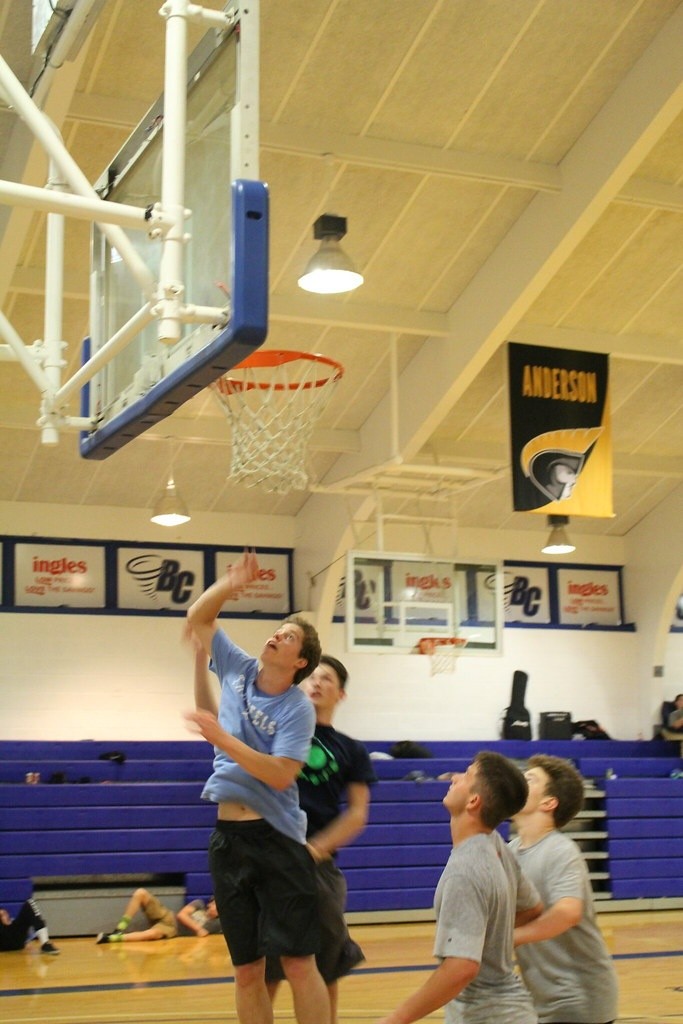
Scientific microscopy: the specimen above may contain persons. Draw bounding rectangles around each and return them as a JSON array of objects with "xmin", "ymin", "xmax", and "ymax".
[
  {"xmin": 191, "ymin": 628, "xmax": 371, "ymax": 1024},
  {"xmin": 187, "ymin": 548, "xmax": 334, "ymax": 1024},
  {"xmin": 382, "ymin": 753, "xmax": 543, "ymax": 1024},
  {"xmin": 95, "ymin": 888, "xmax": 222, "ymax": 943},
  {"xmin": 0, "ymin": 899, "xmax": 60, "ymax": 955},
  {"xmin": 504, "ymin": 755, "xmax": 621, "ymax": 1024}
]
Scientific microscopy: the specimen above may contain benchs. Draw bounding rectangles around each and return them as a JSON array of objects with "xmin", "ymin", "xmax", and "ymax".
[{"xmin": 1, "ymin": 739, "xmax": 683, "ymax": 939}]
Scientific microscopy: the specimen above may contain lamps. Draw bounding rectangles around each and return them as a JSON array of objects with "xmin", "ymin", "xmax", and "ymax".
[
  {"xmin": 296, "ymin": 213, "xmax": 366, "ymax": 296},
  {"xmin": 541, "ymin": 514, "xmax": 577, "ymax": 555},
  {"xmin": 149, "ymin": 435, "xmax": 191, "ymax": 527}
]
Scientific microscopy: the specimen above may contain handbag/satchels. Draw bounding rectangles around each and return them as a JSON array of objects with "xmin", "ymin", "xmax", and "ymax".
[{"xmin": 538, "ymin": 711, "xmax": 573, "ymax": 739}]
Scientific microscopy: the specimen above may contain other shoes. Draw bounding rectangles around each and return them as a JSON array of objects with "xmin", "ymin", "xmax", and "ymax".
[
  {"xmin": 96, "ymin": 932, "xmax": 109, "ymax": 945},
  {"xmin": 110, "ymin": 929, "xmax": 123, "ymax": 935},
  {"xmin": 41, "ymin": 941, "xmax": 60, "ymax": 955}
]
